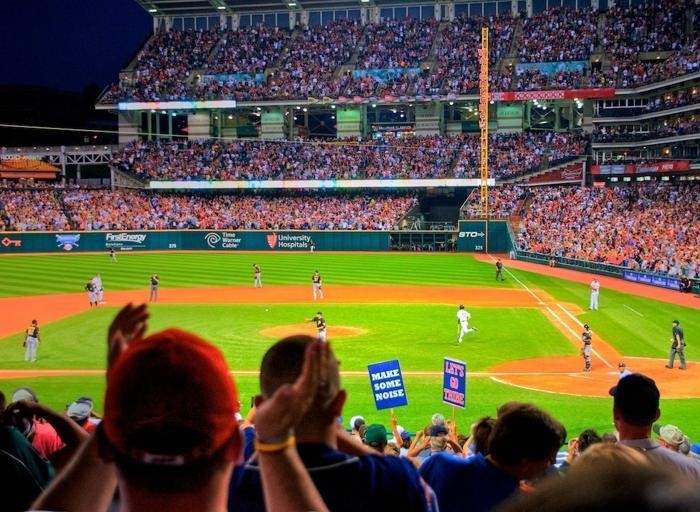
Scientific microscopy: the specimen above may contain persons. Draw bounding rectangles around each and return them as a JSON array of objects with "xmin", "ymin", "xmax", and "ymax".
[
  {"xmin": 312, "ymin": 270, "xmax": 324, "ymax": 300},
  {"xmin": 110, "ymin": 246, "xmax": 118, "ymax": 264},
  {"xmin": 1, "ymin": 177, "xmax": 420, "ymax": 231},
  {"xmin": 309, "ymin": 239, "xmax": 315, "ymax": 252},
  {"xmin": 1, "ymin": 303, "xmax": 700, "ymax": 510},
  {"xmin": 148, "ymin": 271, "xmax": 159, "ymax": 302},
  {"xmin": 448, "ymin": 176, "xmax": 700, "ymax": 311},
  {"xmin": 85, "ymin": 273, "xmax": 105, "ymax": 308},
  {"xmin": 101, "ymin": 1, "xmax": 699, "ymax": 178},
  {"xmin": 252, "ymin": 264, "xmax": 263, "ymax": 289}
]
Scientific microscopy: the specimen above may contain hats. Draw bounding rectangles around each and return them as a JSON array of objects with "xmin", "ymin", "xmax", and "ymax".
[
  {"xmin": 102, "ymin": 330, "xmax": 240, "ymax": 466},
  {"xmin": 608, "ymin": 374, "xmax": 660, "ymax": 410},
  {"xmin": 366, "ymin": 424, "xmax": 387, "ymax": 447},
  {"xmin": 67, "ymin": 398, "xmax": 93, "ymax": 421},
  {"xmin": 426, "ymin": 425, "xmax": 448, "ymax": 436},
  {"xmin": 653, "ymin": 424, "xmax": 685, "ymax": 446}
]
